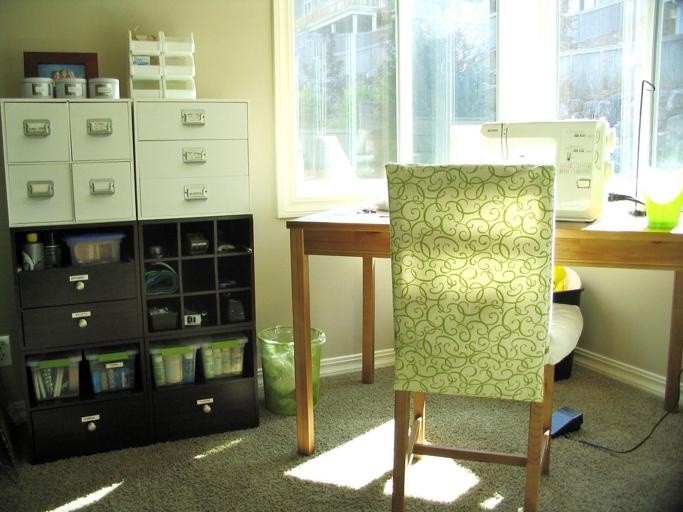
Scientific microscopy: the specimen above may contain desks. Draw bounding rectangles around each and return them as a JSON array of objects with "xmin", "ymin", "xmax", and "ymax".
[{"xmin": 286, "ymin": 211, "xmax": 683, "ymax": 457}]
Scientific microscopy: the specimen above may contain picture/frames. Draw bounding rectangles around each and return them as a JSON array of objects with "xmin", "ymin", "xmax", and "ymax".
[{"xmin": 23, "ymin": 53, "xmax": 99, "ymax": 80}]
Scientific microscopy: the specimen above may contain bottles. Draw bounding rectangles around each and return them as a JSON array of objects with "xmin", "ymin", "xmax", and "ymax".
[
  {"xmin": 151, "ymin": 346, "xmax": 243, "ymax": 386},
  {"xmin": 19, "ymin": 231, "xmax": 46, "ymax": 271}
]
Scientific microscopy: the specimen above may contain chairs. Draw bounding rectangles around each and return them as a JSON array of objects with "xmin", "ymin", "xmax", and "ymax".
[{"xmin": 385, "ymin": 163, "xmax": 555, "ymax": 512}]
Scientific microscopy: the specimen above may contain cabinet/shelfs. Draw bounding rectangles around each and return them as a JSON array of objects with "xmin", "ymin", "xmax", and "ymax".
[{"xmin": 128, "ymin": 30, "xmax": 196, "ymax": 99}]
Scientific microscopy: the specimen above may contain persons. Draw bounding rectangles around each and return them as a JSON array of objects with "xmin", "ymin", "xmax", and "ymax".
[{"xmin": 50, "ymin": 69, "xmax": 77, "ymax": 99}]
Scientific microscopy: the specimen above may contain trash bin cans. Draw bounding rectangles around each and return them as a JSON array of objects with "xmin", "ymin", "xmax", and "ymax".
[{"xmin": 257, "ymin": 326, "xmax": 326, "ymax": 415}]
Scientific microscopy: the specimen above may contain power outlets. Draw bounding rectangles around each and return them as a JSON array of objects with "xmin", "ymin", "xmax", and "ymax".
[{"xmin": 0, "ymin": 336, "xmax": 10, "ymax": 367}]
